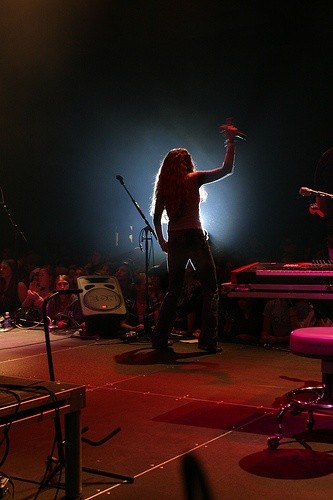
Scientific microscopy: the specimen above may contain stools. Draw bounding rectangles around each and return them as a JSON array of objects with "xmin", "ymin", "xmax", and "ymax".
[{"xmin": 268, "ymin": 326, "xmax": 333, "ymax": 453}]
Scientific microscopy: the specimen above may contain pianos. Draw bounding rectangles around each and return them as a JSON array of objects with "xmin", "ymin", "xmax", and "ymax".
[{"xmin": 220, "ymin": 260, "xmax": 333, "ymax": 325}]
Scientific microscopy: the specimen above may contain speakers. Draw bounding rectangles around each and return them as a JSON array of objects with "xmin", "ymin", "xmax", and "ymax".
[{"xmin": 76, "ymin": 275, "xmax": 128, "ymax": 314}]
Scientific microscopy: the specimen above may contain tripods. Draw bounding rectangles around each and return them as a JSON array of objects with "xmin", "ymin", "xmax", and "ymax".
[
  {"xmin": 121, "ymin": 183, "xmax": 185, "ymax": 340},
  {"xmin": 38, "ymin": 292, "xmax": 135, "ymax": 491}
]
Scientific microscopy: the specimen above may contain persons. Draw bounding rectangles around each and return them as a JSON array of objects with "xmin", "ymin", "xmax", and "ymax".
[
  {"xmin": 151, "ymin": 124, "xmax": 238, "ymax": 355},
  {"xmin": 0, "ymin": 249, "xmax": 333, "ymax": 346}
]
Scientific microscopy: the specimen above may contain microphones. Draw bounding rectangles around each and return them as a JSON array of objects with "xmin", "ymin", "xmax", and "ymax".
[
  {"xmin": 299, "ymin": 187, "xmax": 333, "ymax": 199},
  {"xmin": 235, "ymin": 132, "xmax": 247, "ymax": 141},
  {"xmin": 52, "ymin": 289, "xmax": 84, "ymax": 294},
  {"xmin": 116, "ymin": 175, "xmax": 123, "ymax": 182}
]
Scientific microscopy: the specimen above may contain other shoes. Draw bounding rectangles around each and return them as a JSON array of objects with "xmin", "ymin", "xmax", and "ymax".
[
  {"xmin": 198, "ymin": 345, "xmax": 224, "ymax": 352},
  {"xmin": 152, "ymin": 345, "xmax": 173, "ymax": 353}
]
